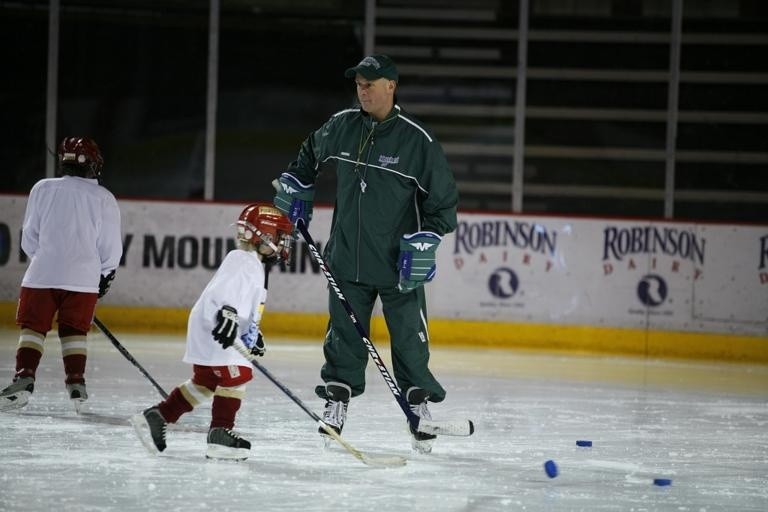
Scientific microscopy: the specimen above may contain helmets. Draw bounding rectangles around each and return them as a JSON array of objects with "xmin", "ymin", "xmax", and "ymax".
[
  {"xmin": 236, "ymin": 202, "xmax": 300, "ymax": 267},
  {"xmin": 56, "ymin": 134, "xmax": 106, "ymax": 179}
]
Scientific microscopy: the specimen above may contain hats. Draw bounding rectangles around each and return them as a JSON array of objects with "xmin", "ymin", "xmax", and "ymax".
[{"xmin": 344, "ymin": 54, "xmax": 402, "ymax": 81}]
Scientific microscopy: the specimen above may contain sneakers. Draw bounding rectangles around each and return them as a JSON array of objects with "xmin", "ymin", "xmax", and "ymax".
[
  {"xmin": 406, "ymin": 386, "xmax": 438, "ymax": 443},
  {"xmin": 0, "ymin": 376, "xmax": 35, "ymax": 396},
  {"xmin": 65, "ymin": 382, "xmax": 88, "ymax": 401},
  {"xmin": 141, "ymin": 404, "xmax": 168, "ymax": 452},
  {"xmin": 207, "ymin": 426, "xmax": 250, "ymax": 448},
  {"xmin": 318, "ymin": 381, "xmax": 352, "ymax": 440}
]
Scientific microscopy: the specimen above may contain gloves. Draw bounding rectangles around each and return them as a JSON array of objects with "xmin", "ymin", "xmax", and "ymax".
[
  {"xmin": 210, "ymin": 304, "xmax": 241, "ymax": 351},
  {"xmin": 271, "ymin": 173, "xmax": 316, "ymax": 242},
  {"xmin": 396, "ymin": 231, "xmax": 442, "ymax": 294},
  {"xmin": 241, "ymin": 329, "xmax": 268, "ymax": 357},
  {"xmin": 97, "ymin": 270, "xmax": 116, "ymax": 300}
]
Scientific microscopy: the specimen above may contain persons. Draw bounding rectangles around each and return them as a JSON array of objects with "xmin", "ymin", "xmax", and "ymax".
[
  {"xmin": 143, "ymin": 201, "xmax": 295, "ymax": 454},
  {"xmin": 272, "ymin": 54, "xmax": 459, "ymax": 441},
  {"xmin": 0, "ymin": 135, "xmax": 123, "ymax": 400}
]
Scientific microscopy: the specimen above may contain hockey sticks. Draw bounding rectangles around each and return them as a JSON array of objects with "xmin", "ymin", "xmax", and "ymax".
[
  {"xmin": 236, "ymin": 339, "xmax": 405, "ymax": 468},
  {"xmin": 295, "ymin": 217, "xmax": 474, "ymax": 438}
]
